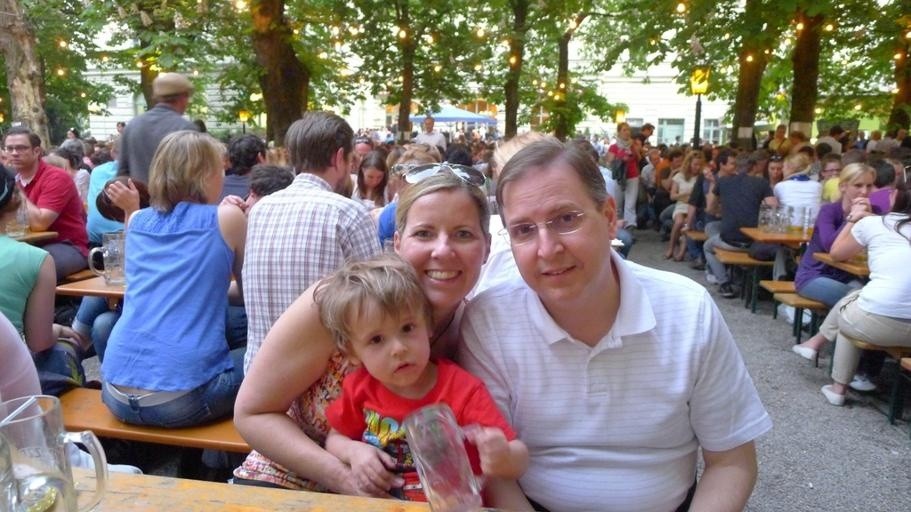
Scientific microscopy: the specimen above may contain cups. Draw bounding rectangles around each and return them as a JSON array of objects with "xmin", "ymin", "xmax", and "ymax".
[
  {"xmin": 0, "ymin": 395, "xmax": 110, "ymax": 512},
  {"xmin": 87, "ymin": 232, "xmax": 126, "ymax": 287},
  {"xmin": 405, "ymin": 400, "xmax": 492, "ymax": 512},
  {"xmin": 759, "ymin": 203, "xmax": 813, "ymax": 235},
  {"xmin": 383, "ymin": 237, "xmax": 395, "ymax": 255},
  {"xmin": 5, "ymin": 200, "xmax": 27, "ymax": 237},
  {"xmin": 855, "ymin": 248, "xmax": 868, "ymax": 264}
]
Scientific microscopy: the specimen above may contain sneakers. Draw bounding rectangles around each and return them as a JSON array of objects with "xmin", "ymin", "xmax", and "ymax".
[
  {"xmin": 822, "ymin": 384, "xmax": 845, "ymax": 407},
  {"xmin": 689, "ymin": 259, "xmax": 706, "ymax": 270},
  {"xmin": 792, "ymin": 344, "xmax": 817, "ymax": 360},
  {"xmin": 719, "ymin": 286, "xmax": 734, "ymax": 297},
  {"xmin": 849, "ymin": 374, "xmax": 877, "ymax": 392}
]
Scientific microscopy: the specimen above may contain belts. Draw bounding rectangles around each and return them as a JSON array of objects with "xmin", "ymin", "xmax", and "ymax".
[{"xmin": 105, "ymin": 381, "xmax": 188, "ymax": 407}]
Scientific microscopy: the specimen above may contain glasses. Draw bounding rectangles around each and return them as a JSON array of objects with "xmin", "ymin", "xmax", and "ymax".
[
  {"xmin": 769, "ymin": 156, "xmax": 784, "ymax": 160},
  {"xmin": 498, "ymin": 206, "xmax": 599, "ymax": 246},
  {"xmin": 403, "ymin": 162, "xmax": 486, "ymax": 188}
]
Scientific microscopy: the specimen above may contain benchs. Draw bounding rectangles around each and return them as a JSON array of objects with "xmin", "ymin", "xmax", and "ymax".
[
  {"xmin": 1, "ymin": 157, "xmax": 621, "ymax": 512},
  {"xmin": 684, "ymin": 188, "xmax": 911, "ymax": 424}
]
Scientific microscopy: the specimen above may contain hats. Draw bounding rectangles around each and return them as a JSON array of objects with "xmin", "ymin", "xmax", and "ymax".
[{"xmin": 151, "ymin": 72, "xmax": 193, "ymax": 97}]
